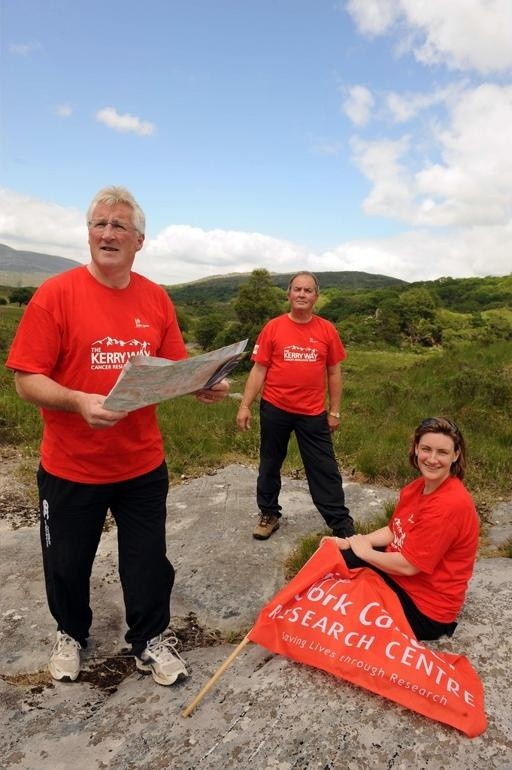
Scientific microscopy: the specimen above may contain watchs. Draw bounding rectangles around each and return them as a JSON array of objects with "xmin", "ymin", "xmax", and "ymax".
[{"xmin": 328, "ymin": 411, "xmax": 340, "ymax": 419}]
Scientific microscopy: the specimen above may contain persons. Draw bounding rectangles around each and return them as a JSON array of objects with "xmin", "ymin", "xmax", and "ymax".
[
  {"xmin": 316, "ymin": 414, "xmax": 482, "ymax": 643},
  {"xmin": 4, "ymin": 182, "xmax": 229, "ymax": 684},
  {"xmin": 234, "ymin": 270, "xmax": 359, "ymax": 543}
]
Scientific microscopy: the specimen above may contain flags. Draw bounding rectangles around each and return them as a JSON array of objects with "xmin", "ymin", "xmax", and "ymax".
[{"xmin": 248, "ymin": 538, "xmax": 488, "ymax": 738}]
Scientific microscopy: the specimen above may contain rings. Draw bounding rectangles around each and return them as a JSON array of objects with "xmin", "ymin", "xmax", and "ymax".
[{"xmin": 214, "ymin": 396, "xmax": 217, "ymax": 401}]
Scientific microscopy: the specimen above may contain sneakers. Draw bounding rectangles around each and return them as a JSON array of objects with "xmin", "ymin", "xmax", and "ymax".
[
  {"xmin": 252, "ymin": 512, "xmax": 281, "ymax": 540},
  {"xmin": 47, "ymin": 631, "xmax": 82, "ymax": 682},
  {"xmin": 135, "ymin": 635, "xmax": 188, "ymax": 686}
]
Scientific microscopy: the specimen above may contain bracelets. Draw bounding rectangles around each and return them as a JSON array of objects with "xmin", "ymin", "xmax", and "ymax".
[{"xmin": 238, "ymin": 406, "xmax": 249, "ymax": 409}]
